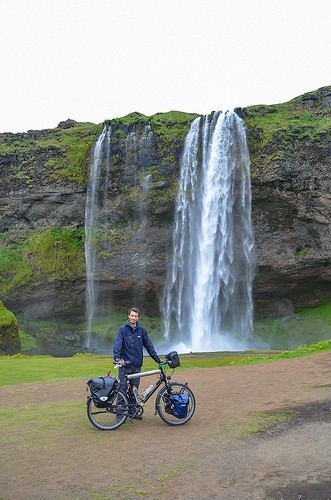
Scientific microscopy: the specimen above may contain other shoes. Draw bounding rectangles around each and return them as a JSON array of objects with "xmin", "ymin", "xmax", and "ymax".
[
  {"xmin": 130, "ymin": 414, "xmax": 142, "ymax": 420},
  {"xmin": 117, "ymin": 417, "xmax": 125, "ymax": 423}
]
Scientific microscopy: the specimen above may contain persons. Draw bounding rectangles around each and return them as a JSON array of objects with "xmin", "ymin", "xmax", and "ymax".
[{"xmin": 113, "ymin": 307, "xmax": 163, "ymax": 422}]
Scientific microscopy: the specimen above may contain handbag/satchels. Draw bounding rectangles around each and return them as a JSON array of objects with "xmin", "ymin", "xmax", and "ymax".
[
  {"xmin": 88, "ymin": 376, "xmax": 117, "ymax": 408},
  {"xmin": 163, "ymin": 392, "xmax": 189, "ymax": 419},
  {"xmin": 165, "ymin": 351, "xmax": 180, "ymax": 368}
]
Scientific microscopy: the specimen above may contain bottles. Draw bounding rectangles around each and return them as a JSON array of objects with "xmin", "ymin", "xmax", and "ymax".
[
  {"xmin": 132, "ymin": 385, "xmax": 142, "ymax": 403},
  {"xmin": 141, "ymin": 384, "xmax": 155, "ymax": 397}
]
